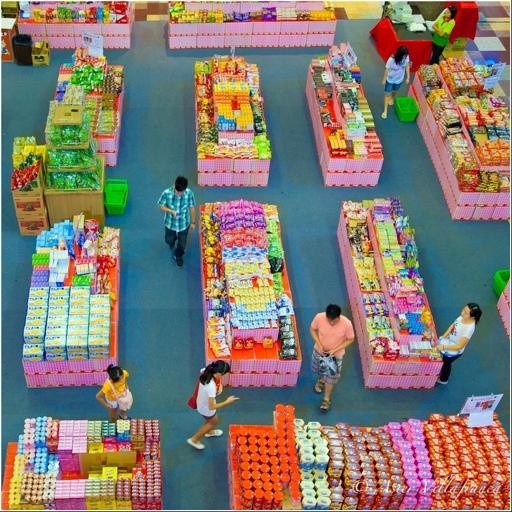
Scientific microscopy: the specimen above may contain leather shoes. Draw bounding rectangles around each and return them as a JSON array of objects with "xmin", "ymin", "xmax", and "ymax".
[
  {"xmin": 176, "ymin": 256, "xmax": 182, "ymax": 265},
  {"xmin": 119, "ymin": 416, "xmax": 131, "ymax": 420}
]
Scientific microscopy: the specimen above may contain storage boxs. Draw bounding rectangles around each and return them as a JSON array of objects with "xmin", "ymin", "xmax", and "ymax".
[{"xmin": 9, "ymin": 135, "xmax": 49, "ymax": 237}]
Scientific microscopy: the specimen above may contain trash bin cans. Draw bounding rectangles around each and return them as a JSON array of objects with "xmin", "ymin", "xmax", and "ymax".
[{"xmin": 13, "ymin": 34, "xmax": 33, "ymax": 65}]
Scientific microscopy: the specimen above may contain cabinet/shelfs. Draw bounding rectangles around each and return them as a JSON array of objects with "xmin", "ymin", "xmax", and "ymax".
[{"xmin": 44, "ymin": 104, "xmax": 107, "ymax": 226}]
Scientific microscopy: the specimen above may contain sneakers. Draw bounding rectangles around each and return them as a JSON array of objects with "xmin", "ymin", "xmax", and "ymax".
[
  {"xmin": 204, "ymin": 429, "xmax": 223, "ymax": 437},
  {"xmin": 186, "ymin": 438, "xmax": 205, "ymax": 450}
]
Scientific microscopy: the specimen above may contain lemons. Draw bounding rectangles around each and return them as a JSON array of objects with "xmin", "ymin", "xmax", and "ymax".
[
  {"xmin": 175, "ymin": 9, "xmax": 224, "ymax": 24},
  {"xmin": 309, "ymin": 8, "xmax": 335, "ymax": 20}
]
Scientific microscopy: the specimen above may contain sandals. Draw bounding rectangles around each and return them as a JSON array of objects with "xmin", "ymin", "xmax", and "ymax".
[
  {"xmin": 320, "ymin": 399, "xmax": 331, "ymax": 413},
  {"xmin": 314, "ymin": 380, "xmax": 325, "ymax": 393}
]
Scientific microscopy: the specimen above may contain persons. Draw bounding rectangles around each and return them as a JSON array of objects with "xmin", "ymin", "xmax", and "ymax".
[
  {"xmin": 430, "ymin": 4, "xmax": 458, "ymax": 65},
  {"xmin": 186, "ymin": 359, "xmax": 240, "ymax": 450},
  {"xmin": 381, "ymin": 45, "xmax": 410, "ymax": 120},
  {"xmin": 309, "ymin": 303, "xmax": 355, "ymax": 414},
  {"xmin": 95, "ymin": 364, "xmax": 134, "ymax": 422},
  {"xmin": 437, "ymin": 302, "xmax": 482, "ymax": 385},
  {"xmin": 156, "ymin": 175, "xmax": 197, "ymax": 268}
]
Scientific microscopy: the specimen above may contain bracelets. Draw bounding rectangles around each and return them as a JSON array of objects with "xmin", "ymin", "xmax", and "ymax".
[
  {"xmin": 191, "ymin": 221, "xmax": 195, "ymax": 224},
  {"xmin": 443, "ymin": 343, "xmax": 447, "ymax": 350}
]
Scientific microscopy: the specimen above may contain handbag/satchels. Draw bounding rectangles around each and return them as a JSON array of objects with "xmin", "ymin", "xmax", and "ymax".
[
  {"xmin": 188, "ymin": 382, "xmax": 199, "ymax": 409},
  {"xmin": 118, "ymin": 389, "xmax": 133, "ymax": 410}
]
